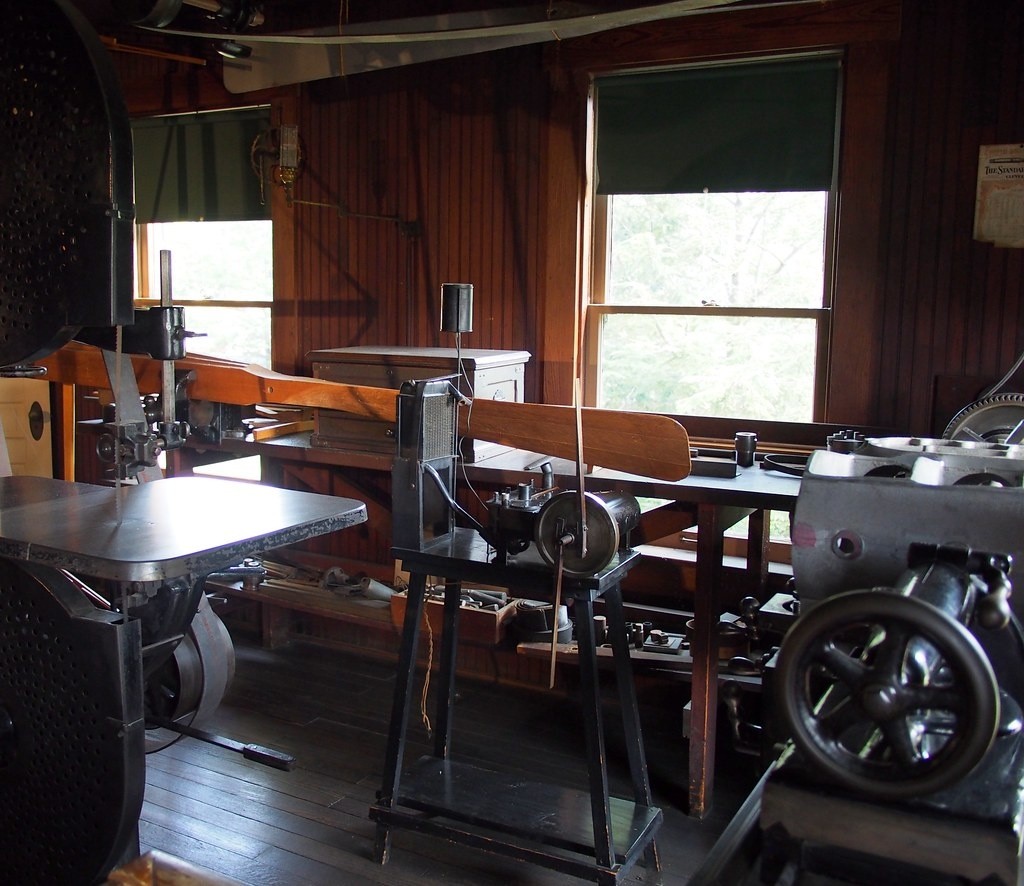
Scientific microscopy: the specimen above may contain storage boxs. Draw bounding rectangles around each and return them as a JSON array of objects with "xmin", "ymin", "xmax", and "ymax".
[{"xmin": 390, "ymin": 586, "xmax": 518, "ymax": 644}]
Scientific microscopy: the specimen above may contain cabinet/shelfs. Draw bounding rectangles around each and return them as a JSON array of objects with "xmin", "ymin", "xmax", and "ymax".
[{"xmin": 307, "ymin": 345, "xmax": 532, "ymax": 465}]
